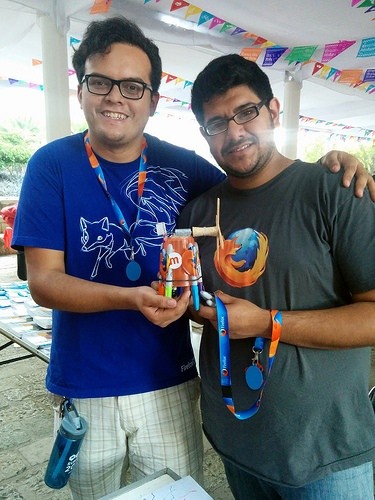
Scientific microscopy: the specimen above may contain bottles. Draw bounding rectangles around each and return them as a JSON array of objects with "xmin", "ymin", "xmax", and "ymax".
[{"xmin": 44, "ymin": 402, "xmax": 88, "ymax": 490}]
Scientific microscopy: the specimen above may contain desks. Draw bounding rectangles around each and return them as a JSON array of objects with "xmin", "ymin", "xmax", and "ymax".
[{"xmin": 0, "ymin": 282, "xmax": 202, "ymax": 378}]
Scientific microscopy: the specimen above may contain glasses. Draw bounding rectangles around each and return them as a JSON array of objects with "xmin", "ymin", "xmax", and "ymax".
[
  {"xmin": 201, "ymin": 97, "xmax": 269, "ymax": 137},
  {"xmin": 81, "ymin": 74, "xmax": 153, "ymax": 100}
]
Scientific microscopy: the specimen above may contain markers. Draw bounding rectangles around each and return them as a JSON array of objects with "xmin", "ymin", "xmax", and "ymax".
[
  {"xmin": 158, "ymin": 242, "xmax": 173, "ymax": 299},
  {"xmin": 187, "ymin": 243, "xmax": 204, "ymax": 312},
  {"xmin": 199, "ymin": 289, "xmax": 216, "ymax": 307}
]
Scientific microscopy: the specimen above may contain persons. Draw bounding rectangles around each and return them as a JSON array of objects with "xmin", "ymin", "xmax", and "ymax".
[
  {"xmin": 10, "ymin": 17, "xmax": 374, "ymax": 500},
  {"xmin": 148, "ymin": 55, "xmax": 375, "ymax": 500}
]
